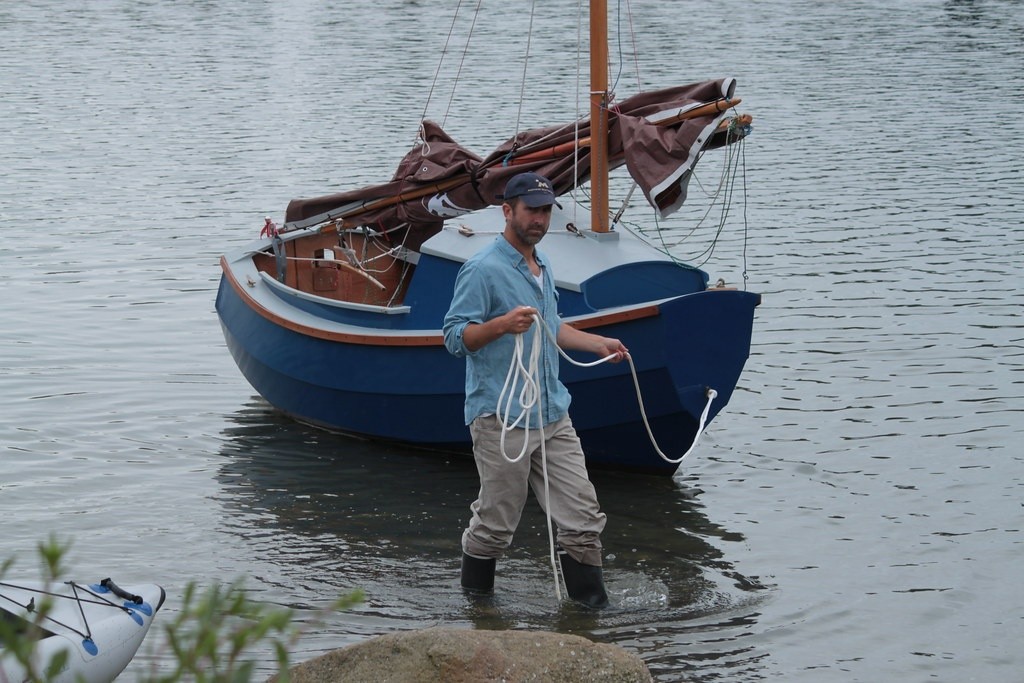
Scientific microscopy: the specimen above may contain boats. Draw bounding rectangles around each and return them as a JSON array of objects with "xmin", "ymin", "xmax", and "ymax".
[{"xmin": 214, "ymin": 0, "xmax": 765, "ymax": 483}]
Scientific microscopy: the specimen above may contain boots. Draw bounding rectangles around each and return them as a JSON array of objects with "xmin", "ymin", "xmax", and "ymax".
[
  {"xmin": 556, "ymin": 545, "xmax": 610, "ymax": 610},
  {"xmin": 459, "ymin": 552, "xmax": 497, "ymax": 590}
]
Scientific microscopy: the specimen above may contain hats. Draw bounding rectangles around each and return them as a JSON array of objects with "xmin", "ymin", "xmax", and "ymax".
[{"xmin": 504, "ymin": 171, "xmax": 563, "ymax": 211}]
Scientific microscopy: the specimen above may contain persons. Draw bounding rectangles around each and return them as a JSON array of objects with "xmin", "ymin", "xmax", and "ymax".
[{"xmin": 439, "ymin": 168, "xmax": 638, "ymax": 623}]
